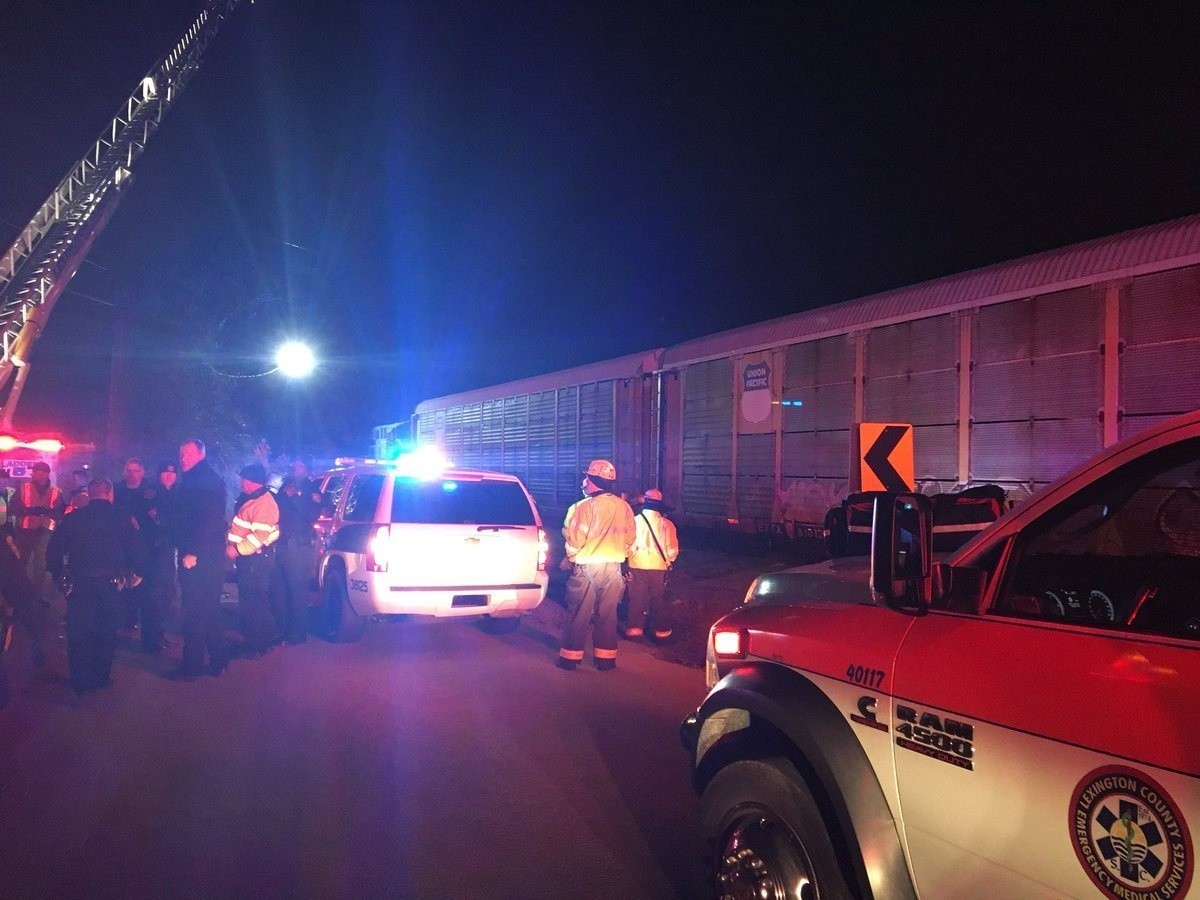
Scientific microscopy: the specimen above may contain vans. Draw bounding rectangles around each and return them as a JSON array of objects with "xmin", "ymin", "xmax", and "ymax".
[{"xmin": 310, "ymin": 456, "xmax": 551, "ymax": 644}]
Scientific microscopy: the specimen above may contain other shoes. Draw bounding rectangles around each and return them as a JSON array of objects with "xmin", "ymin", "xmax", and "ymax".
[
  {"xmin": 141, "ymin": 644, "xmax": 168, "ymax": 652},
  {"xmin": 274, "ymin": 635, "xmax": 308, "ymax": 645},
  {"xmin": 208, "ymin": 663, "xmax": 226, "ymax": 677},
  {"xmin": 169, "ymin": 667, "xmax": 196, "ymax": 681},
  {"xmin": 597, "ymin": 661, "xmax": 612, "ymax": 671},
  {"xmin": 556, "ymin": 659, "xmax": 576, "ymax": 669},
  {"xmin": 162, "ymin": 640, "xmax": 179, "ymax": 646}
]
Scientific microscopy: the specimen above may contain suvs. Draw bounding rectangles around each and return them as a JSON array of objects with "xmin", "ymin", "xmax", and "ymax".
[{"xmin": 679, "ymin": 407, "xmax": 1200, "ymax": 900}]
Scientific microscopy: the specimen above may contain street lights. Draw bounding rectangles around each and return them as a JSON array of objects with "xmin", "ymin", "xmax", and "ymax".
[{"xmin": 274, "ymin": 336, "xmax": 317, "ymax": 464}]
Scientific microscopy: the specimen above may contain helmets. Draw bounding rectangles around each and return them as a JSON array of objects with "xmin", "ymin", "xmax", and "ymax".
[
  {"xmin": 582, "ymin": 460, "xmax": 616, "ymax": 480},
  {"xmin": 644, "ymin": 488, "xmax": 662, "ymax": 501}
]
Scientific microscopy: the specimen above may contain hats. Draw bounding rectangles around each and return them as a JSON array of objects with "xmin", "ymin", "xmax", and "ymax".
[
  {"xmin": 240, "ymin": 463, "xmax": 266, "ymax": 483},
  {"xmin": 32, "ymin": 462, "xmax": 50, "ymax": 473}
]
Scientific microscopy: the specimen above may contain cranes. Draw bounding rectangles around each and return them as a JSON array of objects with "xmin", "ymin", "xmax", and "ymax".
[{"xmin": 0, "ymin": 0, "xmax": 236, "ymax": 441}]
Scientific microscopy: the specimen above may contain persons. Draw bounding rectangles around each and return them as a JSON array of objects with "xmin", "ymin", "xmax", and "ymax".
[
  {"xmin": 255, "ymin": 438, "xmax": 272, "ymax": 471},
  {"xmin": 158, "ymin": 438, "xmax": 229, "ymax": 683},
  {"xmin": 223, "ymin": 461, "xmax": 280, "ymax": 659},
  {"xmin": 624, "ymin": 489, "xmax": 679, "ymax": 643},
  {"xmin": 267, "ymin": 454, "xmax": 333, "ymax": 650},
  {"xmin": 555, "ymin": 460, "xmax": 637, "ymax": 670},
  {"xmin": 0, "ymin": 447, "xmax": 179, "ymax": 695}
]
